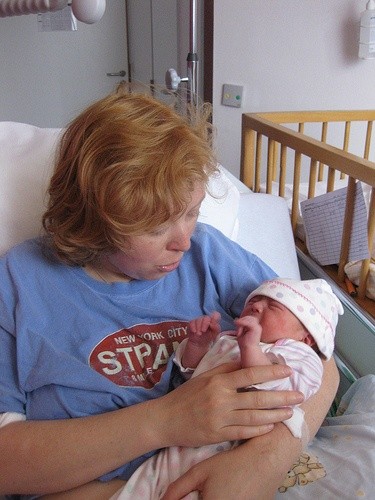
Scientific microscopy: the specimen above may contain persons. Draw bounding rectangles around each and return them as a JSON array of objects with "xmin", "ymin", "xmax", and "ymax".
[
  {"xmin": 0, "ymin": 79, "xmax": 340, "ymax": 500},
  {"xmin": 108, "ymin": 277, "xmax": 345, "ymax": 500}
]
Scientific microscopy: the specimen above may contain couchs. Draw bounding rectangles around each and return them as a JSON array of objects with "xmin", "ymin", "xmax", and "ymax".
[{"xmin": 0, "ymin": 119, "xmax": 375, "ymax": 418}]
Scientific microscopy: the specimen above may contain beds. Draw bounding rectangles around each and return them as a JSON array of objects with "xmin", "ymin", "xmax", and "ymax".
[{"xmin": 238, "ymin": 108, "xmax": 375, "ymax": 387}]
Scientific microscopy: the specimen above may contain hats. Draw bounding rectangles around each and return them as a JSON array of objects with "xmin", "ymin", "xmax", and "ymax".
[{"xmin": 244, "ymin": 278, "xmax": 344, "ymax": 361}]
